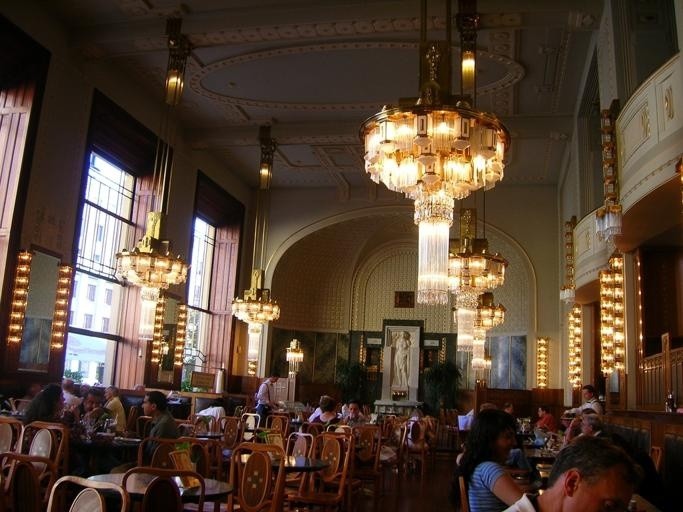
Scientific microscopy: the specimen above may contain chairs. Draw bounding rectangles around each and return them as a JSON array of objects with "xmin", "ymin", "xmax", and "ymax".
[{"xmin": 647, "ymin": 445, "xmax": 664, "ymax": 473}]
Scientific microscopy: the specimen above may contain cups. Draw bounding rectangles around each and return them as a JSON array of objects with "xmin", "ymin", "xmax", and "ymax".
[
  {"xmin": 341, "ymin": 411, "xmax": 384, "ymax": 430},
  {"xmin": 76, "ymin": 414, "xmax": 119, "ymax": 441}
]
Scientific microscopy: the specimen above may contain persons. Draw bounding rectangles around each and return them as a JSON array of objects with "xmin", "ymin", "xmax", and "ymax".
[
  {"xmin": 457, "ymin": 409, "xmax": 537, "ymax": 511},
  {"xmin": 12, "ymin": 378, "xmax": 616, "ymax": 476},
  {"xmin": 504, "ymin": 435, "xmax": 647, "ymax": 512},
  {"xmin": 393, "ymin": 330, "xmax": 412, "ymax": 387},
  {"xmin": 254, "ymin": 371, "xmax": 280, "ymax": 419}
]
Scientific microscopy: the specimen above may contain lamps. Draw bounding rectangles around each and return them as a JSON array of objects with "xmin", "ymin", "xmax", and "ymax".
[
  {"xmin": 114, "ymin": 36, "xmax": 190, "ymax": 343},
  {"xmin": 229, "ymin": 129, "xmax": 280, "ymax": 363}
]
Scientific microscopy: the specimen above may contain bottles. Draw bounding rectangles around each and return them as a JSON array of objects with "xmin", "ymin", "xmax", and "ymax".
[{"xmin": 664, "ymin": 393, "xmax": 674, "ymax": 413}]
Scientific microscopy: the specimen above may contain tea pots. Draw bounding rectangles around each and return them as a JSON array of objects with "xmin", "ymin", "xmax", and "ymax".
[
  {"xmin": 520, "ymin": 418, "xmax": 531, "ymax": 434},
  {"xmin": 533, "ymin": 430, "xmax": 549, "ymax": 446}
]
